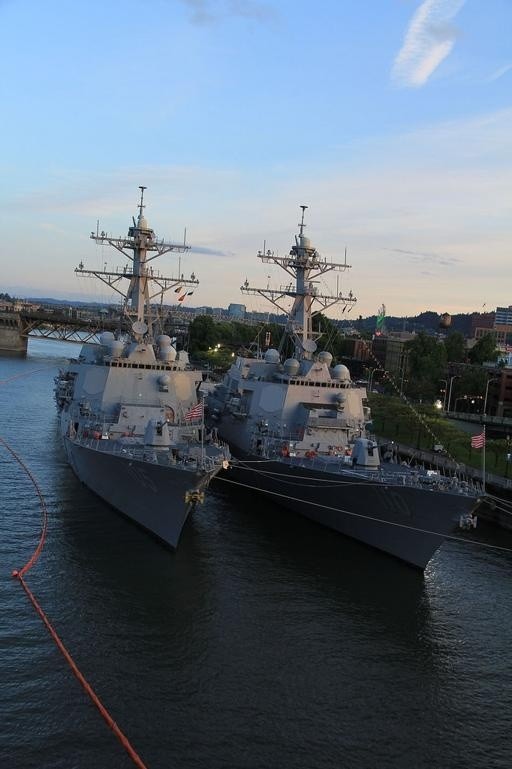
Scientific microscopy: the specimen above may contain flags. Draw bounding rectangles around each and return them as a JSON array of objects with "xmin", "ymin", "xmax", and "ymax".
[
  {"xmin": 184, "ymin": 402, "xmax": 202, "ymax": 420},
  {"xmin": 471, "ymin": 433, "xmax": 485, "ymax": 449}
]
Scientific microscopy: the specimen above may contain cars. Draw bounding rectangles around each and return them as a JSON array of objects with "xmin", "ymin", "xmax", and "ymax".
[{"xmin": 79, "ymin": 318, "xmax": 92, "ymax": 323}]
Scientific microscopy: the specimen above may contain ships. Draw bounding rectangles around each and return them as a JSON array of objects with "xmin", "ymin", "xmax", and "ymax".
[
  {"xmin": 200, "ymin": 206, "xmax": 489, "ymax": 575},
  {"xmin": 55, "ymin": 185, "xmax": 232, "ymax": 551}
]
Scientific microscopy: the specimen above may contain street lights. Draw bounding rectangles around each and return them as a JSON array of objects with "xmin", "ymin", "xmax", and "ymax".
[
  {"xmin": 359, "ymin": 365, "xmax": 404, "ymax": 398},
  {"xmin": 437, "ymin": 375, "xmax": 498, "ymax": 417}
]
